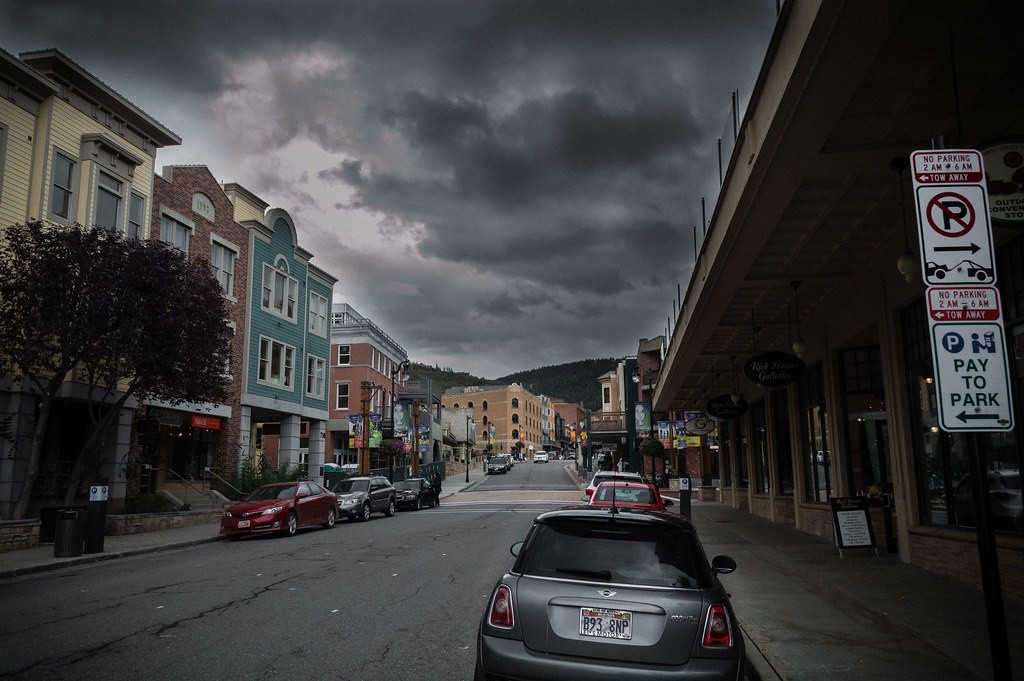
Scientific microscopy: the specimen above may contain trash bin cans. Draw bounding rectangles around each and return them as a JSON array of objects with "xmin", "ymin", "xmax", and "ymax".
[{"xmin": 54, "ymin": 506, "xmax": 85, "ymax": 557}]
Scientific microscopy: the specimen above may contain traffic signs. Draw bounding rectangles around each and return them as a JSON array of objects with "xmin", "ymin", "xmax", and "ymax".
[
  {"xmin": 929, "ymin": 285, "xmax": 1016, "ymax": 437},
  {"xmin": 910, "ymin": 146, "xmax": 1000, "ymax": 285}
]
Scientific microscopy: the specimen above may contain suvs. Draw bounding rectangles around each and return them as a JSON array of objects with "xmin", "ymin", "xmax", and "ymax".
[
  {"xmin": 584, "ymin": 470, "xmax": 648, "ymax": 501},
  {"xmin": 329, "ymin": 473, "xmax": 397, "ymax": 522}
]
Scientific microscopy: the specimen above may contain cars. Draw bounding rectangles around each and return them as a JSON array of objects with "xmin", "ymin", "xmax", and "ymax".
[
  {"xmin": 548, "ymin": 452, "xmax": 575, "ymax": 459},
  {"xmin": 474, "ymin": 504, "xmax": 747, "ymax": 681},
  {"xmin": 532, "ymin": 450, "xmax": 549, "ymax": 464},
  {"xmin": 389, "ymin": 477, "xmax": 439, "ymax": 510},
  {"xmin": 581, "ymin": 480, "xmax": 675, "ymax": 513},
  {"xmin": 218, "ymin": 479, "xmax": 340, "ymax": 543},
  {"xmin": 486, "ymin": 453, "xmax": 514, "ymax": 475}
]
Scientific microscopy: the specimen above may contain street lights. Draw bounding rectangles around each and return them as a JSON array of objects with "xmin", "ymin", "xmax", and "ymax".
[
  {"xmin": 464, "ymin": 415, "xmax": 476, "ymax": 483},
  {"xmin": 389, "ymin": 359, "xmax": 411, "ymax": 485}
]
